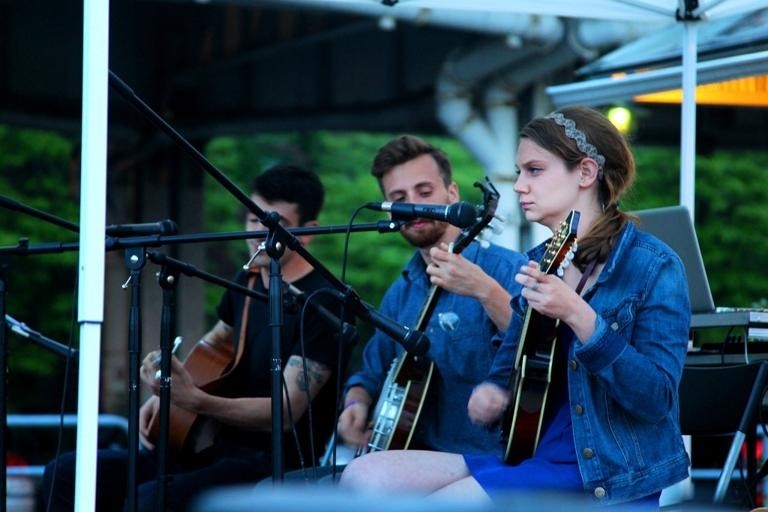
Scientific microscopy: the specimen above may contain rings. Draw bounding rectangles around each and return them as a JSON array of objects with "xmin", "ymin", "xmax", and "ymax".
[{"xmin": 531, "ymin": 281, "xmax": 540, "ymax": 290}]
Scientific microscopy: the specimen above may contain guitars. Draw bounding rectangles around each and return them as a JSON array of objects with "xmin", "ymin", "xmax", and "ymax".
[
  {"xmin": 147, "ymin": 320, "xmax": 235, "ymax": 447},
  {"xmin": 357, "ymin": 175, "xmax": 504, "ymax": 457},
  {"xmin": 504, "ymin": 210, "xmax": 581, "ymax": 465}
]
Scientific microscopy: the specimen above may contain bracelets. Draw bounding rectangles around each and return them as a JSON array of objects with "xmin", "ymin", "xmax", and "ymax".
[{"xmin": 344, "ymin": 399, "xmax": 367, "ymax": 408}]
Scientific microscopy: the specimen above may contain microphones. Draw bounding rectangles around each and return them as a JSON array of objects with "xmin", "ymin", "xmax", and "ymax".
[
  {"xmin": 337, "ymin": 291, "xmax": 430, "ymax": 356},
  {"xmin": 106, "ymin": 220, "xmax": 177, "ymax": 242},
  {"xmin": 5, "ymin": 313, "xmax": 78, "ymax": 362},
  {"xmin": 366, "ymin": 200, "xmax": 479, "ymax": 228}
]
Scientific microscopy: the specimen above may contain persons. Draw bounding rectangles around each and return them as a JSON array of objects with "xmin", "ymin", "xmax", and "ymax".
[
  {"xmin": 339, "ymin": 107, "xmax": 691, "ymax": 512},
  {"xmin": 44, "ymin": 164, "xmax": 356, "ymax": 512},
  {"xmin": 252, "ymin": 135, "xmax": 530, "ymax": 496}
]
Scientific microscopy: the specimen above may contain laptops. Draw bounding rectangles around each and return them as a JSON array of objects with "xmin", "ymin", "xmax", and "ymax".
[{"xmin": 621, "ymin": 205, "xmax": 768, "ymax": 313}]
